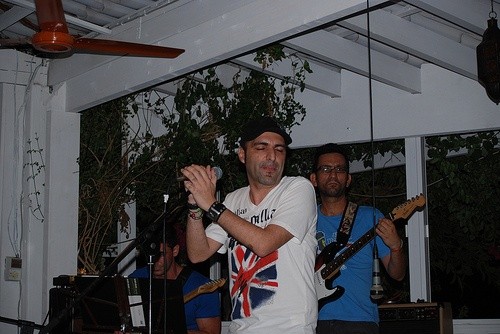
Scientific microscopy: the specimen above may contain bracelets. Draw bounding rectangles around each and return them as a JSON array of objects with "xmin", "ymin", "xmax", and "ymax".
[
  {"xmin": 187, "ymin": 202, "xmax": 205, "ymax": 220},
  {"xmin": 390, "ymin": 238, "xmax": 404, "ymax": 252},
  {"xmin": 206, "ymin": 200, "xmax": 227, "ymax": 223}
]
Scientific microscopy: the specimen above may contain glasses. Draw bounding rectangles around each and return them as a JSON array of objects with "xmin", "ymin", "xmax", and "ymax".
[{"xmin": 317, "ymin": 166, "xmax": 348, "ymax": 173}]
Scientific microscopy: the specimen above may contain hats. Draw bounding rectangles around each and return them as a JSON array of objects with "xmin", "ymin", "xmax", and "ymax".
[{"xmin": 243, "ymin": 116, "xmax": 292, "ymax": 144}]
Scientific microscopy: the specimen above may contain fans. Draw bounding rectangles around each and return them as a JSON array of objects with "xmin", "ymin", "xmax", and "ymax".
[{"xmin": 0, "ymin": 0, "xmax": 185, "ymax": 58}]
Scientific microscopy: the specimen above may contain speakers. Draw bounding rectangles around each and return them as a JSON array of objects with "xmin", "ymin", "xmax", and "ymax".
[{"xmin": 378, "ymin": 303, "xmax": 453, "ymax": 334}]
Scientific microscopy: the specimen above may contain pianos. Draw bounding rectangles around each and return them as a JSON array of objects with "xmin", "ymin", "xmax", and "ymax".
[{"xmin": 48, "ymin": 274, "xmax": 188, "ymax": 334}]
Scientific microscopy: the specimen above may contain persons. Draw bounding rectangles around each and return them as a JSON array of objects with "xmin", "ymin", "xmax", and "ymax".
[
  {"xmin": 180, "ymin": 116, "xmax": 318, "ymax": 334},
  {"xmin": 309, "ymin": 142, "xmax": 407, "ymax": 334},
  {"xmin": 126, "ymin": 223, "xmax": 222, "ymax": 334}
]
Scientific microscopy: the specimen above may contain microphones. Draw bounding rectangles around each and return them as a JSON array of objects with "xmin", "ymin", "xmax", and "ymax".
[
  {"xmin": 370, "ymin": 244, "xmax": 384, "ymax": 299},
  {"xmin": 212, "ymin": 167, "xmax": 223, "ymax": 179}
]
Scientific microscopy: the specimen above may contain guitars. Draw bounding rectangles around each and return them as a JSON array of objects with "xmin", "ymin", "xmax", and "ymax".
[
  {"xmin": 184, "ymin": 278, "xmax": 226, "ymax": 303},
  {"xmin": 314, "ymin": 192, "xmax": 426, "ymax": 313}
]
